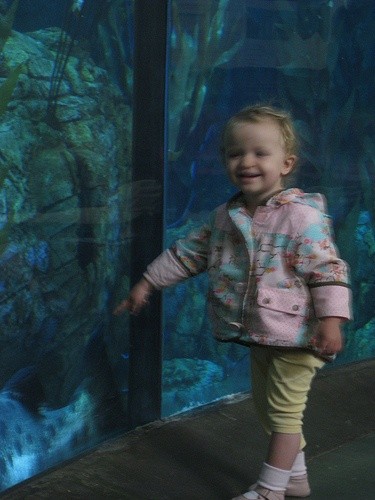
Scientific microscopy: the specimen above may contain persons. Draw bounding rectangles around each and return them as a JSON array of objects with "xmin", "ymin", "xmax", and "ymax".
[{"xmin": 110, "ymin": 103, "xmax": 352, "ymax": 499}]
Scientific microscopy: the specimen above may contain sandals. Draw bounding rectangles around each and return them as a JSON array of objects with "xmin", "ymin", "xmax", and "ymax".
[
  {"xmin": 247, "ymin": 474, "xmax": 310, "ymax": 498},
  {"xmin": 231, "ymin": 485, "xmax": 285, "ymax": 500}
]
